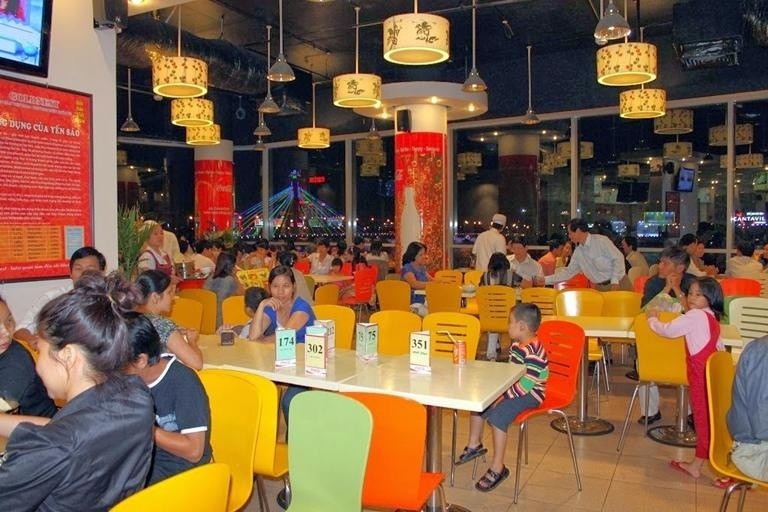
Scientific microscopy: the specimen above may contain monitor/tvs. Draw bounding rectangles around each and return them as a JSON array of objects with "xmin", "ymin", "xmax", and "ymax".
[
  {"xmin": 674, "ymin": 166, "xmax": 695, "ymax": 192},
  {"xmin": 0, "ymin": 0, "xmax": 53, "ymax": 78}
]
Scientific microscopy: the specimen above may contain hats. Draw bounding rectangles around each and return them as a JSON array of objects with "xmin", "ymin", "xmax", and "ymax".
[
  {"xmin": 492, "ymin": 214, "xmax": 507, "ymax": 226},
  {"xmin": 138, "ymin": 219, "xmax": 158, "ymax": 233}
]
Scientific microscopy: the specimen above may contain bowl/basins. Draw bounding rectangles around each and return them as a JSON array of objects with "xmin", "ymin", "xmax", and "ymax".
[{"xmin": 463, "ymin": 285, "xmax": 476, "ymax": 293}]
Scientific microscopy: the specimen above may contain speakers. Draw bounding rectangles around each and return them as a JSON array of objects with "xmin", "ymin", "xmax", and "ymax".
[
  {"xmin": 397, "ymin": 109, "xmax": 412, "ymax": 133},
  {"xmin": 93, "ymin": 0, "xmax": 128, "ymax": 33}
]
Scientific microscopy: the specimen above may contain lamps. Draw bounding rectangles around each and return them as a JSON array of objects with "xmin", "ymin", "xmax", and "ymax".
[
  {"xmin": 520, "ymin": 46, "xmax": 540, "ymax": 125},
  {"xmin": 119, "ymin": 150, "xmax": 168, "ymax": 204},
  {"xmin": 613, "ymin": 109, "xmax": 768, "ymax": 177},
  {"xmin": 333, "ymin": 8, "xmax": 381, "ymax": 108},
  {"xmin": 462, "ymin": 1, "xmax": 486, "ymax": 93},
  {"xmin": 122, "ymin": 5, "xmax": 222, "ymax": 148},
  {"xmin": 357, "ymin": 130, "xmax": 593, "ymax": 202},
  {"xmin": 298, "ymin": 85, "xmax": 331, "ymax": 149},
  {"xmin": 252, "ymin": 0, "xmax": 295, "ymax": 139},
  {"xmin": 598, "ymin": 3, "xmax": 666, "ymax": 122},
  {"xmin": 383, "ymin": 0, "xmax": 450, "ymax": 66}
]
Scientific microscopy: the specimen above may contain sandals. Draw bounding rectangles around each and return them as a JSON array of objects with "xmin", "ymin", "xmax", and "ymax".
[
  {"xmin": 474, "ymin": 464, "xmax": 510, "ymax": 492},
  {"xmin": 454, "ymin": 444, "xmax": 489, "ymax": 465}
]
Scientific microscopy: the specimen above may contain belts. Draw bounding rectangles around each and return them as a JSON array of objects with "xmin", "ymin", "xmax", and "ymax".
[{"xmin": 597, "ymin": 280, "xmax": 610, "ymax": 286}]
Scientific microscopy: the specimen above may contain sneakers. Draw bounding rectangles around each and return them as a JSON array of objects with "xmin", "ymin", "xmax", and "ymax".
[
  {"xmin": 637, "ymin": 410, "xmax": 662, "ymax": 426},
  {"xmin": 688, "ymin": 414, "xmax": 694, "ymax": 428}
]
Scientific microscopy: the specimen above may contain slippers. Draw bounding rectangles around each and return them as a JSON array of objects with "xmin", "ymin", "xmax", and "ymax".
[
  {"xmin": 712, "ymin": 477, "xmax": 752, "ymax": 491},
  {"xmin": 670, "ymin": 459, "xmax": 701, "ymax": 479}
]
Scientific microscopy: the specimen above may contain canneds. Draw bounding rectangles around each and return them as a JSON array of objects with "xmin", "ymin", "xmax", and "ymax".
[{"xmin": 452, "ymin": 340, "xmax": 467, "ymax": 365}]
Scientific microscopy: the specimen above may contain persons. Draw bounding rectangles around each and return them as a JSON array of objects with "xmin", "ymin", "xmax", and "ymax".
[
  {"xmin": 453, "ymin": 302, "xmax": 549, "ymax": 492},
  {"xmin": 645, "ymin": 275, "xmax": 754, "ymax": 491},
  {"xmin": 635, "ymin": 248, "xmax": 701, "ymax": 426},
  {"xmin": 725, "ymin": 334, "xmax": 768, "ymax": 484},
  {"xmin": 399, "ymin": 215, "xmax": 767, "ymax": 315},
  {"xmin": 1, "ymin": 214, "xmax": 390, "ymax": 511}
]
